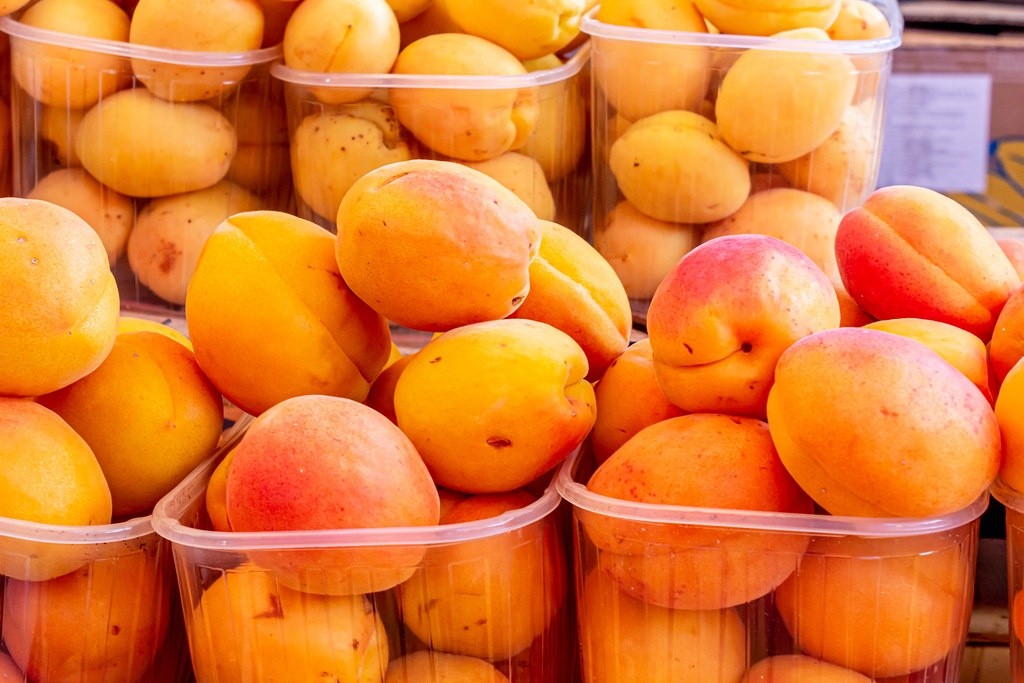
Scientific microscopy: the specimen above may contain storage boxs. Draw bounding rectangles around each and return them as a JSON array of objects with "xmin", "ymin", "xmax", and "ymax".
[
  {"xmin": 150, "ymin": 407, "xmax": 575, "ymax": 683},
  {"xmin": 557, "ymin": 435, "xmax": 990, "ymax": 683},
  {"xmin": 0, "ymin": 402, "xmax": 250, "ymax": 683},
  {"xmin": 270, "ymin": 38, "xmax": 590, "ymax": 240},
  {"xmin": 0, "ymin": 14, "xmax": 285, "ymax": 321},
  {"xmin": 575, "ymin": 0, "xmax": 913, "ymax": 322},
  {"xmin": 973, "ymin": 468, "xmax": 1024, "ymax": 683}
]
